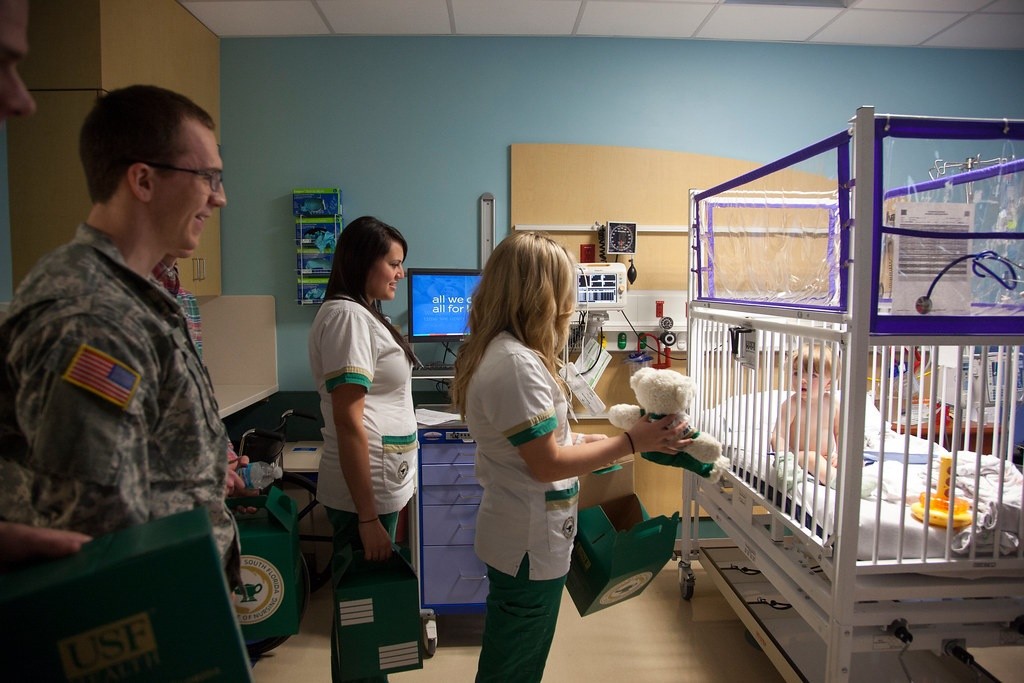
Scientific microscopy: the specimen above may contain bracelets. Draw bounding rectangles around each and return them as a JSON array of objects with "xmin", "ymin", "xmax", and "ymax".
[
  {"xmin": 624, "ymin": 431, "xmax": 635, "ymax": 454},
  {"xmin": 576, "ymin": 434, "xmax": 583, "ymax": 445},
  {"xmin": 360, "ymin": 518, "xmax": 378, "ymax": 524}
]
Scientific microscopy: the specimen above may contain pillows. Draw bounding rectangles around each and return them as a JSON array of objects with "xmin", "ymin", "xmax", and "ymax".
[{"xmin": 713, "ymin": 389, "xmax": 891, "ymax": 436}]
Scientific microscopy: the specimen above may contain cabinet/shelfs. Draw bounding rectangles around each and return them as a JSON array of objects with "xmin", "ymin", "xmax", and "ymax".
[
  {"xmin": 410, "ymin": 425, "xmax": 495, "ymax": 614},
  {"xmin": 12, "ymin": 2, "xmax": 225, "ymax": 313}
]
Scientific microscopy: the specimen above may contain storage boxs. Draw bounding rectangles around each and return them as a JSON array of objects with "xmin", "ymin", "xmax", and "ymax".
[{"xmin": 567, "ymin": 460, "xmax": 681, "ymax": 620}]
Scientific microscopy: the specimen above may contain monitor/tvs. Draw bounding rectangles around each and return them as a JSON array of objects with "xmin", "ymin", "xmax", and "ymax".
[{"xmin": 406, "ymin": 268, "xmax": 484, "ymax": 343}]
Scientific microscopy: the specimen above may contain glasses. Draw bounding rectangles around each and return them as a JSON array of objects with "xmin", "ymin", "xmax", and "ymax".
[{"xmin": 128, "ymin": 160, "xmax": 222, "ymax": 192}]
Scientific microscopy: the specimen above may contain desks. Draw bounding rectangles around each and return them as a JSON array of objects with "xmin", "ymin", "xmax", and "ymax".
[
  {"xmin": 283, "ymin": 437, "xmax": 336, "ymax": 549},
  {"xmin": 210, "ymin": 382, "xmax": 279, "ymax": 420}
]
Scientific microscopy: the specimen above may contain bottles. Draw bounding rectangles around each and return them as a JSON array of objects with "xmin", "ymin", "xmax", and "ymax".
[{"xmin": 235, "ymin": 461, "xmax": 283, "ymax": 490}]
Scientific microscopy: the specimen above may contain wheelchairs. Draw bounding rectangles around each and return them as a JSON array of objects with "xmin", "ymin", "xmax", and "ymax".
[{"xmin": 232, "ymin": 407, "xmax": 341, "ymax": 596}]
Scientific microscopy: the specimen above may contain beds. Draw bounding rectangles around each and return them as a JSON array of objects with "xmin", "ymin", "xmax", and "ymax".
[{"xmin": 679, "ymin": 300, "xmax": 1022, "ymax": 682}]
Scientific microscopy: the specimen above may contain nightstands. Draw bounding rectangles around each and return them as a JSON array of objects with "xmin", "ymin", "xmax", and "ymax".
[{"xmin": 890, "ymin": 412, "xmax": 1009, "ymax": 458}]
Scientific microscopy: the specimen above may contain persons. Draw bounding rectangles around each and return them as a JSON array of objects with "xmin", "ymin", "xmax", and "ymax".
[
  {"xmin": 771, "ymin": 344, "xmax": 874, "ymax": 497},
  {"xmin": 455, "ymin": 232, "xmax": 692, "ymax": 683},
  {"xmin": 309, "ymin": 216, "xmax": 418, "ymax": 683},
  {"xmin": 0, "ymin": 1, "xmax": 260, "ymax": 683}
]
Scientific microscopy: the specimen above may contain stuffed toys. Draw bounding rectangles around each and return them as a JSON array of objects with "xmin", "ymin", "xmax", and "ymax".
[{"xmin": 608, "ymin": 366, "xmax": 729, "ymax": 480}]
